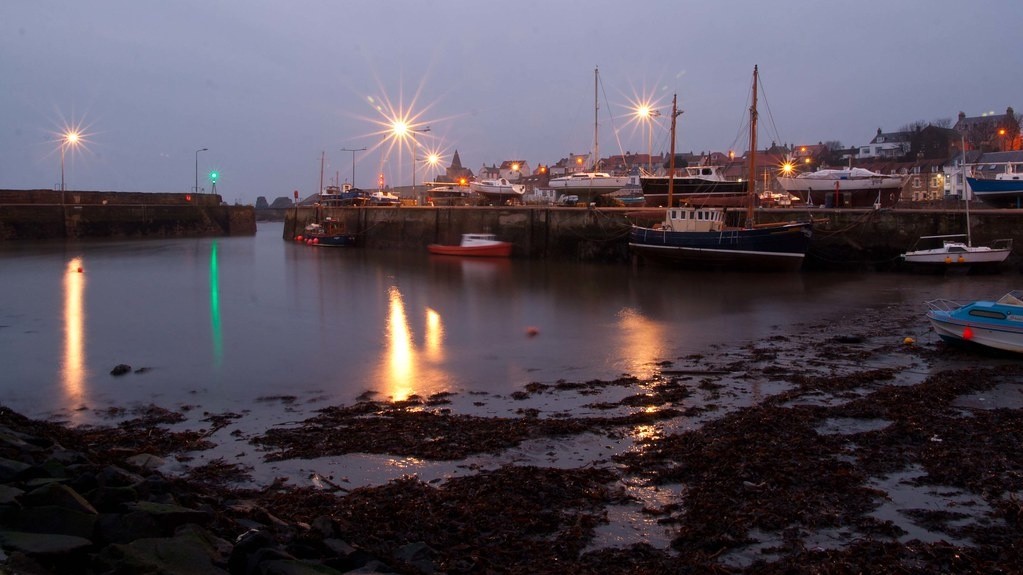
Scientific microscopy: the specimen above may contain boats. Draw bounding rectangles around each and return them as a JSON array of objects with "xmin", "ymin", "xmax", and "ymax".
[
  {"xmin": 776, "ymin": 153, "xmax": 912, "ymax": 200},
  {"xmin": 427, "ymin": 232, "xmax": 514, "ymax": 258},
  {"xmin": 426, "ymin": 182, "xmax": 474, "ymax": 198},
  {"xmin": 924, "ymin": 288, "xmax": 1023, "ymax": 355},
  {"xmin": 964, "ymin": 162, "xmax": 1022, "ymax": 198},
  {"xmin": 638, "ymin": 149, "xmax": 759, "ymax": 207},
  {"xmin": 468, "ymin": 176, "xmax": 527, "ymax": 197}
]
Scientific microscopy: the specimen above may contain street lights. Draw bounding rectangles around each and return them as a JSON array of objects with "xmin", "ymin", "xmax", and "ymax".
[
  {"xmin": 61, "ymin": 128, "xmax": 81, "ymax": 238},
  {"xmin": 428, "ymin": 152, "xmax": 440, "ymax": 181},
  {"xmin": 196, "ymin": 148, "xmax": 209, "ymax": 193},
  {"xmin": 637, "ymin": 105, "xmax": 653, "ymax": 175},
  {"xmin": 393, "ymin": 121, "xmax": 416, "ymax": 198}
]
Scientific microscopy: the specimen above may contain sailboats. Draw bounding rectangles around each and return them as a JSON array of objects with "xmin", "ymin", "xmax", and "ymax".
[
  {"xmin": 295, "ymin": 215, "xmax": 362, "ymax": 247},
  {"xmin": 626, "ymin": 63, "xmax": 814, "ymax": 270},
  {"xmin": 900, "ymin": 134, "xmax": 1015, "ymax": 264},
  {"xmin": 547, "ymin": 63, "xmax": 629, "ymax": 202},
  {"xmin": 318, "ymin": 171, "xmax": 401, "ymax": 207}
]
